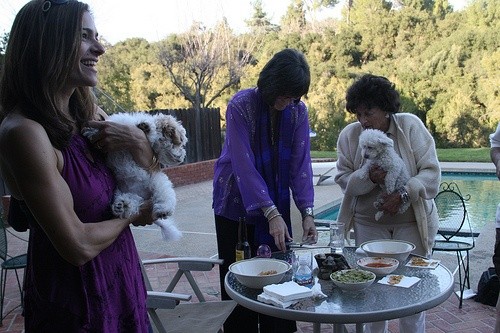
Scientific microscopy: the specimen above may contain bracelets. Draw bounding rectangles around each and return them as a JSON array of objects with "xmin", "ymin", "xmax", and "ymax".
[
  {"xmin": 145, "ymin": 156, "xmax": 159, "ymax": 170},
  {"xmin": 265, "ymin": 207, "xmax": 277, "ymax": 218},
  {"xmin": 301, "ymin": 208, "xmax": 314, "ymax": 220},
  {"xmin": 268, "ymin": 214, "xmax": 282, "ymax": 222},
  {"xmin": 264, "ymin": 205, "xmax": 275, "ymax": 216}
]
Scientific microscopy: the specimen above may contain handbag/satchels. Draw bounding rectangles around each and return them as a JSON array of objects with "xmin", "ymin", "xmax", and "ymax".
[{"xmin": 472, "ymin": 266, "xmax": 500, "ymax": 307}]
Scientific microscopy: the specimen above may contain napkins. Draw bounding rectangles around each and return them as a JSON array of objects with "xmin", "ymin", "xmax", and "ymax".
[
  {"xmin": 406, "ymin": 257, "xmax": 441, "ymax": 269},
  {"xmin": 377, "ymin": 273, "xmax": 421, "ymax": 288},
  {"xmin": 257, "ymin": 279, "xmax": 329, "ymax": 308}
]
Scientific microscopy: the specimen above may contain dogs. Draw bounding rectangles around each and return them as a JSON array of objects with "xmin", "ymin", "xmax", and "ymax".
[
  {"xmin": 357, "ymin": 127, "xmax": 416, "ymax": 221},
  {"xmin": 81, "ymin": 109, "xmax": 188, "ymax": 245}
]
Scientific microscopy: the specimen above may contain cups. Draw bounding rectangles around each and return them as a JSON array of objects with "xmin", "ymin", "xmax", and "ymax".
[
  {"xmin": 292, "ymin": 249, "xmax": 313, "ymax": 286},
  {"xmin": 329, "ymin": 221, "xmax": 345, "ymax": 250}
]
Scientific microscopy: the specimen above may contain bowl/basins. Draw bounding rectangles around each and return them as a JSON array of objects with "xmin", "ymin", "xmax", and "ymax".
[
  {"xmin": 357, "ymin": 256, "xmax": 399, "ymax": 277},
  {"xmin": 228, "ymin": 257, "xmax": 291, "ymax": 289},
  {"xmin": 329, "ymin": 269, "xmax": 377, "ymax": 294},
  {"xmin": 363, "ymin": 239, "xmax": 416, "ymax": 267}
]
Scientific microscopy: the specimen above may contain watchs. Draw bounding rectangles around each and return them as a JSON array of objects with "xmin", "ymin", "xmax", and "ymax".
[{"xmin": 397, "ymin": 186, "xmax": 409, "ymax": 204}]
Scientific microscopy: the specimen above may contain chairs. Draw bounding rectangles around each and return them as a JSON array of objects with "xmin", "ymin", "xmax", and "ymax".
[
  {"xmin": 142, "ymin": 257, "xmax": 239, "ymax": 333},
  {"xmin": 0, "ymin": 195, "xmax": 29, "ymax": 329},
  {"xmin": 425, "ymin": 180, "xmax": 479, "ymax": 309}
]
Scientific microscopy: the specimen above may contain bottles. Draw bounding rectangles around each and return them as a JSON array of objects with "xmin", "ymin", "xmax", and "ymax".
[
  {"xmin": 257, "ymin": 239, "xmax": 271, "ymax": 258},
  {"xmin": 234, "ymin": 216, "xmax": 251, "ymax": 261}
]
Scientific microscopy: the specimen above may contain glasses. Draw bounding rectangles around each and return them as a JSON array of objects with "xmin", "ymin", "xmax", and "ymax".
[{"xmin": 279, "ymin": 96, "xmax": 300, "ymax": 102}]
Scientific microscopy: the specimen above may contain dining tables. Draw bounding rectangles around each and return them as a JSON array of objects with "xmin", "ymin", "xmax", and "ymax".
[{"xmin": 226, "ymin": 245, "xmax": 455, "ymax": 333}]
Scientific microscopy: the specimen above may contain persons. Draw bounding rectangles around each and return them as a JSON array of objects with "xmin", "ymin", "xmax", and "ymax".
[
  {"xmin": 334, "ymin": 74, "xmax": 442, "ymax": 333},
  {"xmin": 0, "ymin": 0, "xmax": 156, "ymax": 333},
  {"xmin": 489, "ymin": 122, "xmax": 500, "ymax": 266},
  {"xmin": 211, "ymin": 48, "xmax": 318, "ymax": 333}
]
